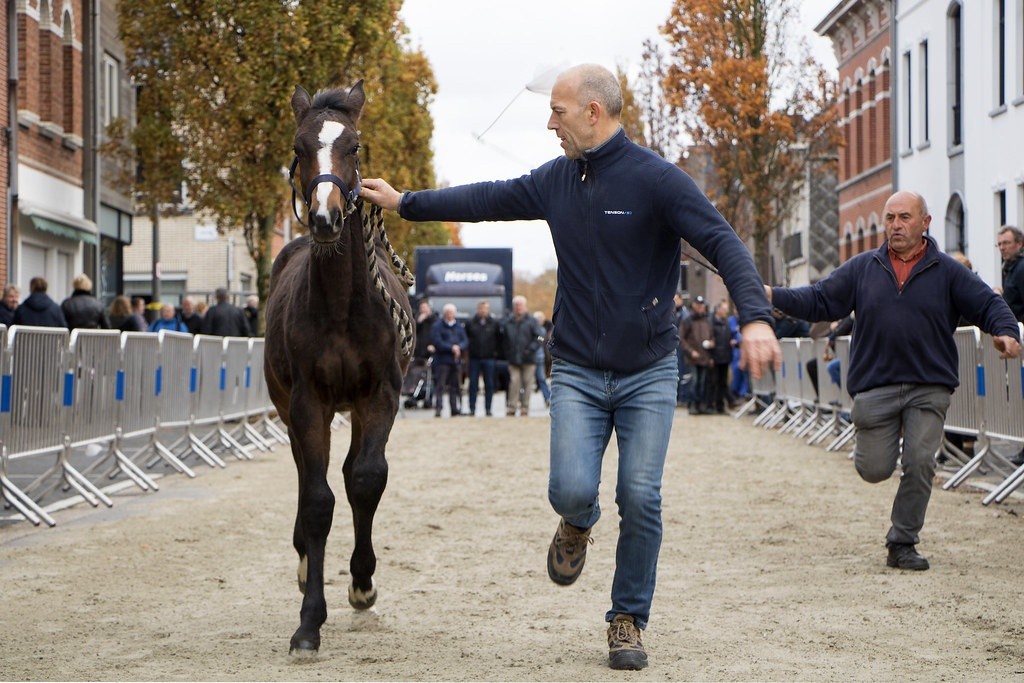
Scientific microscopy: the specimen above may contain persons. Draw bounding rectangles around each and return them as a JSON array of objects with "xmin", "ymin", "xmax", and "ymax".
[
  {"xmin": 948, "ymin": 224, "xmax": 1024, "ymax": 327},
  {"xmin": 766, "ymin": 189, "xmax": 1024, "ymax": 569},
  {"xmin": 674, "ymin": 291, "xmax": 855, "ymax": 415},
  {"xmin": 0, "ymin": 274, "xmax": 260, "ymax": 337},
  {"xmin": 356, "ymin": 64, "xmax": 781, "ymax": 669},
  {"xmin": 405, "ymin": 299, "xmax": 553, "ymax": 417}
]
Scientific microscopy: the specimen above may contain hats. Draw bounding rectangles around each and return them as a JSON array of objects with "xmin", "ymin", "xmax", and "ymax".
[
  {"xmin": 694, "ymin": 296, "xmax": 704, "ymax": 303},
  {"xmin": 73, "ymin": 273, "xmax": 92, "ymax": 291}
]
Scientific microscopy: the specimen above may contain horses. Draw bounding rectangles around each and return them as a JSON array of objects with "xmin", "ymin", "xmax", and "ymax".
[{"xmin": 263, "ymin": 78, "xmax": 417, "ymax": 656}]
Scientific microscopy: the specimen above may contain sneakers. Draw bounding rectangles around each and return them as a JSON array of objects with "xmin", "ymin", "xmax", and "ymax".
[
  {"xmin": 607, "ymin": 614, "xmax": 647, "ymax": 669},
  {"xmin": 547, "ymin": 517, "xmax": 595, "ymax": 585},
  {"xmin": 887, "ymin": 543, "xmax": 930, "ymax": 570}
]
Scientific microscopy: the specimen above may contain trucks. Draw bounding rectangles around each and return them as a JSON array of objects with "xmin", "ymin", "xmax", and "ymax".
[{"xmin": 417, "ymin": 246, "xmax": 516, "ymax": 321}]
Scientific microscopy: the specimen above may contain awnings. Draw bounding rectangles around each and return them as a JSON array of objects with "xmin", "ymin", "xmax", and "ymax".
[{"xmin": 20, "ymin": 200, "xmax": 99, "ymax": 245}]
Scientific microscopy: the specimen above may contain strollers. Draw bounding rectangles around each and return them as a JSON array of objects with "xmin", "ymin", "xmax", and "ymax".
[{"xmin": 401, "ymin": 348, "xmax": 439, "ymax": 411}]
